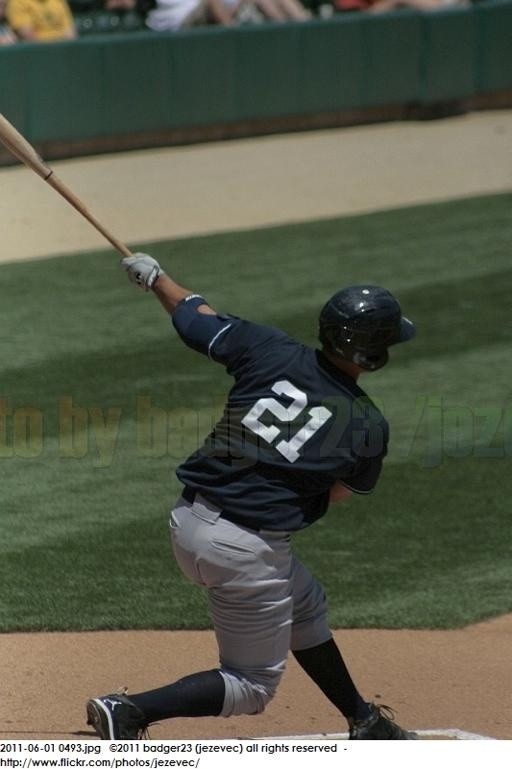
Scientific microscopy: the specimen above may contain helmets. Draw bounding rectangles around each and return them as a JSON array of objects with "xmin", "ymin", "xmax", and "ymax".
[{"xmin": 314, "ymin": 282, "xmax": 422, "ymax": 373}]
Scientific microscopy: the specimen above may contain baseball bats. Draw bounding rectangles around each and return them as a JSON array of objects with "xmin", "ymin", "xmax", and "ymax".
[{"xmin": 1, "ymin": 113, "xmax": 132, "ymax": 257}]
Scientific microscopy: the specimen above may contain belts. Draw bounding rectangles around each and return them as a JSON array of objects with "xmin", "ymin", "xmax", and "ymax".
[{"xmin": 177, "ymin": 482, "xmax": 295, "ymax": 540}]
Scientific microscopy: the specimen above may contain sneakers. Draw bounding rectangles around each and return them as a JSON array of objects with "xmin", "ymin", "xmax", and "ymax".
[
  {"xmin": 87, "ymin": 688, "xmax": 150, "ymax": 740},
  {"xmin": 347, "ymin": 702, "xmax": 421, "ymax": 740}
]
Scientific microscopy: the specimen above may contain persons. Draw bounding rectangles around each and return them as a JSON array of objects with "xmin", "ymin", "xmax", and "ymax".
[
  {"xmin": 88, "ymin": 253, "xmax": 420, "ymax": 739},
  {"xmin": 0, "ymin": 0, "xmax": 473, "ymax": 45}
]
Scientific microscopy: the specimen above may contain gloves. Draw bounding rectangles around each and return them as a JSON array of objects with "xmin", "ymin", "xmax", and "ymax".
[{"xmin": 121, "ymin": 248, "xmax": 165, "ymax": 294}]
